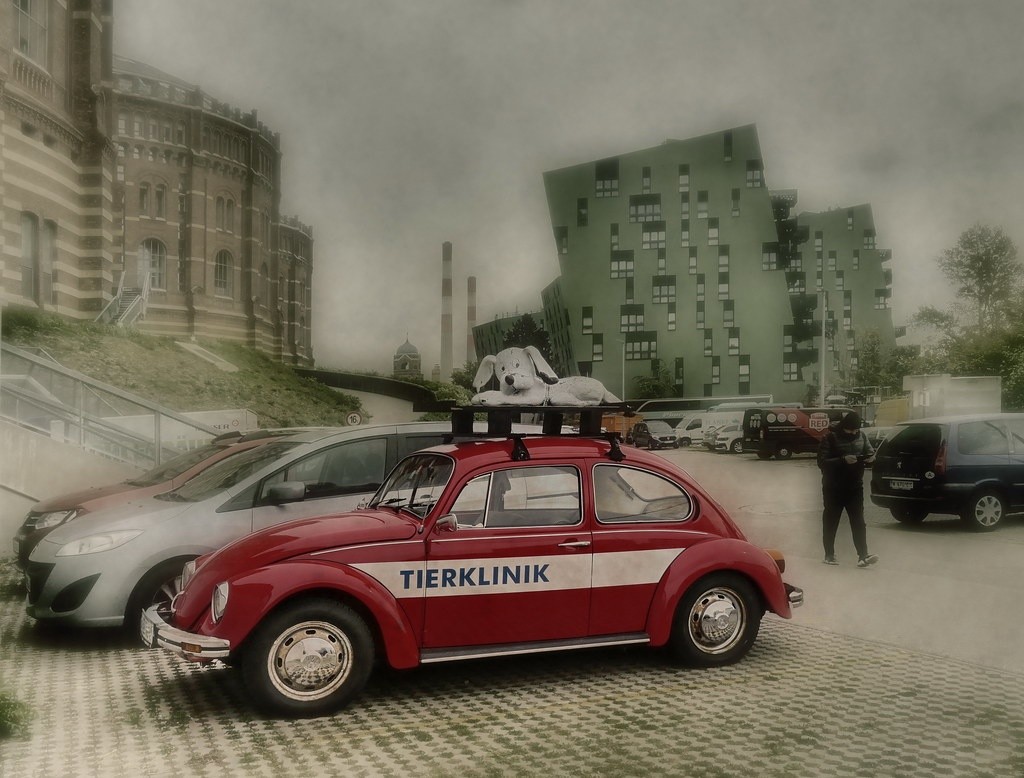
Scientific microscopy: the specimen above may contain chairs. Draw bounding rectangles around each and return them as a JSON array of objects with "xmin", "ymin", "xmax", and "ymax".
[{"xmin": 474, "ymin": 510, "xmax": 523, "ymax": 526}]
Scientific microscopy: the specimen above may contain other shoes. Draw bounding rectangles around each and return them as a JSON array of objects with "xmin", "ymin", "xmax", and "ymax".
[
  {"xmin": 857, "ymin": 555, "xmax": 879, "ymax": 567},
  {"xmin": 824, "ymin": 554, "xmax": 840, "ymax": 565}
]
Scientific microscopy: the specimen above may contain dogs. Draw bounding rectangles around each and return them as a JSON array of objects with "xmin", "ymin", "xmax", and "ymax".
[{"xmin": 470, "ymin": 346, "xmax": 632, "ymax": 408}]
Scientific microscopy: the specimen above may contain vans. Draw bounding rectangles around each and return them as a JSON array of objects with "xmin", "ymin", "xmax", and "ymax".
[
  {"xmin": 703, "ymin": 401, "xmax": 878, "ymax": 460},
  {"xmin": 673, "ymin": 413, "xmax": 704, "ymax": 447}
]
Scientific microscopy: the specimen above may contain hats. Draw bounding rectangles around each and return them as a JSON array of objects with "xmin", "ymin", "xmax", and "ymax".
[{"xmin": 842, "ymin": 411, "xmax": 862, "ymax": 429}]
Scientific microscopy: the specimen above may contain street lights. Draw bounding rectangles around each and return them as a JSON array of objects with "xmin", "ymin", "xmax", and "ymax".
[
  {"xmin": 616, "ymin": 338, "xmax": 624, "ymax": 401},
  {"xmin": 817, "ymin": 287, "xmax": 826, "ymax": 409}
]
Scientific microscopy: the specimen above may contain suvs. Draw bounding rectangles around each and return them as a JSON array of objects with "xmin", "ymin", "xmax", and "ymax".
[{"xmin": 631, "ymin": 420, "xmax": 681, "ymax": 451}]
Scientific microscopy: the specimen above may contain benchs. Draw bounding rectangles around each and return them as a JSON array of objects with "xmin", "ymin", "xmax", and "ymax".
[{"xmin": 569, "ymin": 510, "xmax": 657, "ymax": 523}]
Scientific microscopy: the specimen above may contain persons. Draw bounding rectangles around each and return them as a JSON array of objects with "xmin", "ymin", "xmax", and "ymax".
[{"xmin": 817, "ymin": 411, "xmax": 879, "ymax": 567}]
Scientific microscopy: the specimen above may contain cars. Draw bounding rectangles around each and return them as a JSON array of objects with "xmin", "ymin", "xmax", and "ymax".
[
  {"xmin": 869, "ymin": 414, "xmax": 1024, "ymax": 533},
  {"xmin": 11, "ymin": 402, "xmax": 805, "ymax": 720}
]
